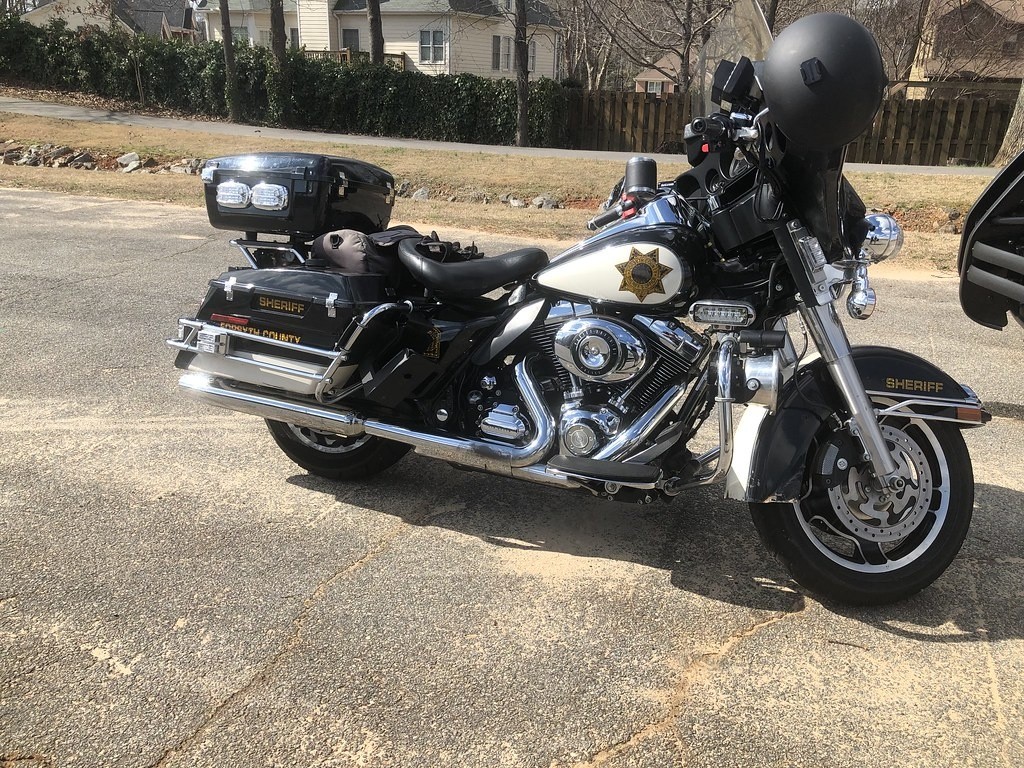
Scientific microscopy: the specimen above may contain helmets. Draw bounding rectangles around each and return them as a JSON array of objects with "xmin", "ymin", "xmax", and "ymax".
[{"xmin": 762, "ymin": 9, "xmax": 889, "ymax": 154}]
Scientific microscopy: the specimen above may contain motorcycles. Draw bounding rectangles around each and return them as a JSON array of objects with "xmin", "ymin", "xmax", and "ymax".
[{"xmin": 164, "ymin": 55, "xmax": 992, "ymax": 606}]
